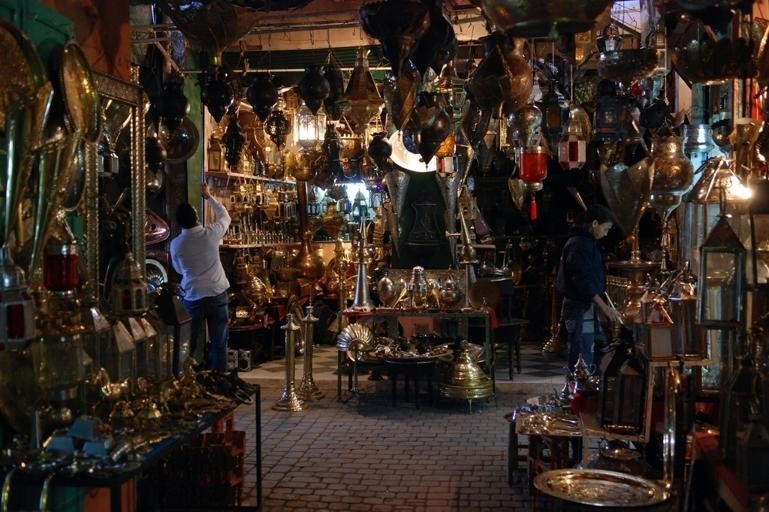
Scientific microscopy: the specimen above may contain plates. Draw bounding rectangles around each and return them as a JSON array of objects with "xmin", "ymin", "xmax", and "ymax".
[
  {"xmin": 61, "ymin": 41, "xmax": 102, "ymax": 142},
  {"xmin": 0, "ymin": 16, "xmax": 48, "ymax": 155},
  {"xmin": 62, "ymin": 144, "xmax": 87, "ymax": 214}
]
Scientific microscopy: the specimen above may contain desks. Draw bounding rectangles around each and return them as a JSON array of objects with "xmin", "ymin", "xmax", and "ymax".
[
  {"xmin": 0, "ymin": 383, "xmax": 262, "ymax": 512},
  {"xmin": 338, "ymin": 308, "xmax": 493, "ymax": 404}
]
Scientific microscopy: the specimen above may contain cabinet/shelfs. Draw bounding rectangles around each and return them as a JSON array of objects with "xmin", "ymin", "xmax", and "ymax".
[{"xmin": 203, "ymin": 98, "xmax": 302, "ymax": 249}]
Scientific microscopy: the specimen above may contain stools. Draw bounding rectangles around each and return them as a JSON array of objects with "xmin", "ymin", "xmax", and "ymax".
[
  {"xmin": 508, "ymin": 422, "xmax": 528, "ymax": 487},
  {"xmin": 494, "ymin": 327, "xmax": 521, "ymax": 380},
  {"xmin": 528, "ymin": 436, "xmax": 583, "ymax": 497},
  {"xmin": 599, "ymin": 369, "xmax": 644, "ymax": 434}
]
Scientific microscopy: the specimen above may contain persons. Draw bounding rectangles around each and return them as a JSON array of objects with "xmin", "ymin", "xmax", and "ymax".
[
  {"xmin": 169, "ymin": 182, "xmax": 231, "ymax": 374},
  {"xmin": 558, "ymin": 204, "xmax": 621, "ymax": 380}
]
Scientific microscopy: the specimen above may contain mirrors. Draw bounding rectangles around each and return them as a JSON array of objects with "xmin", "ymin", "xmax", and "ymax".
[{"xmin": 85, "ymin": 71, "xmax": 146, "ymax": 321}]
